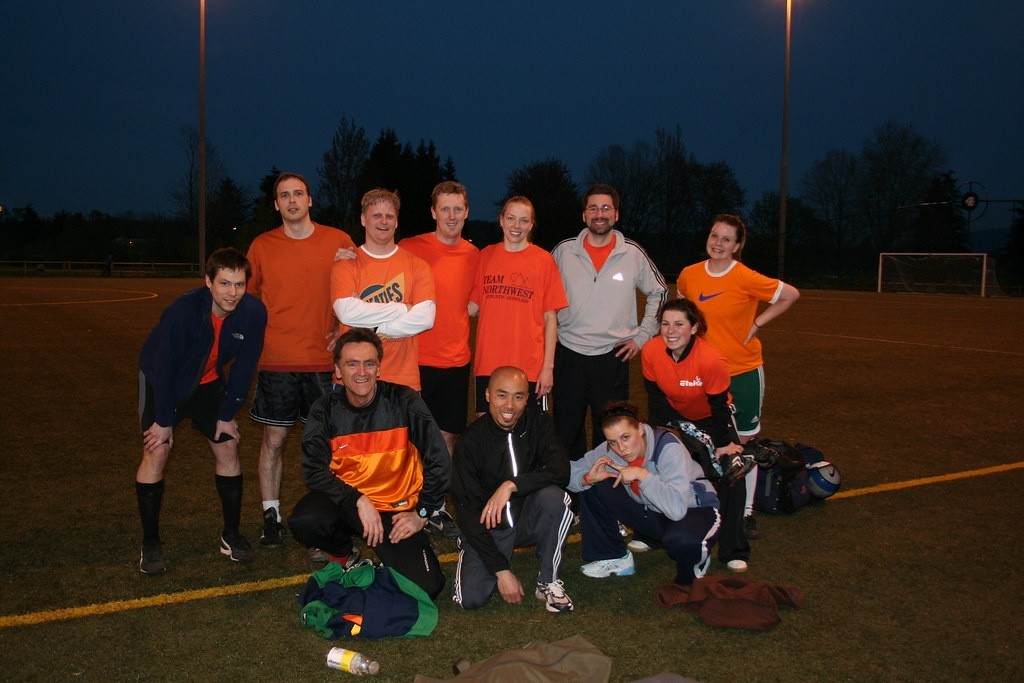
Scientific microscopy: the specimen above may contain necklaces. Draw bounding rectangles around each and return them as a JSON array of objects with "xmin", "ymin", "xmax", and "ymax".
[{"xmin": 362, "ymin": 388, "xmax": 375, "ymax": 407}]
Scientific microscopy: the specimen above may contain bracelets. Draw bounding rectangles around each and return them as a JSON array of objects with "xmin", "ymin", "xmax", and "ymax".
[
  {"xmin": 584, "ymin": 472, "xmax": 595, "ymax": 485},
  {"xmin": 754, "ymin": 318, "xmax": 762, "ymax": 328}
]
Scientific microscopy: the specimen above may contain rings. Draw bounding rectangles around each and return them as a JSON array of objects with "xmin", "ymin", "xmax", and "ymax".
[{"xmin": 488, "ymin": 509, "xmax": 493, "ymax": 512}]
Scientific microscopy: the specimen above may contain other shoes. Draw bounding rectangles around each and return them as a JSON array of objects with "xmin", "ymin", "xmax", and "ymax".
[
  {"xmin": 628, "ymin": 538, "xmax": 654, "ymax": 554},
  {"xmin": 727, "ymin": 559, "xmax": 747, "ymax": 573},
  {"xmin": 328, "ymin": 547, "xmax": 360, "ymax": 570}
]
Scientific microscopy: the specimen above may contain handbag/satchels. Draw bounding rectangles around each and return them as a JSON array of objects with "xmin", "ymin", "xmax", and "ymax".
[{"xmin": 749, "ymin": 438, "xmax": 810, "ymax": 513}]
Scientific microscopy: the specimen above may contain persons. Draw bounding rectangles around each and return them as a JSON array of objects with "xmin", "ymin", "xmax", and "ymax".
[
  {"xmin": 468, "ymin": 197, "xmax": 569, "ymax": 417},
  {"xmin": 329, "ymin": 189, "xmax": 436, "ymax": 399},
  {"xmin": 334, "ymin": 182, "xmax": 479, "ymax": 537},
  {"xmin": 678, "ymin": 215, "xmax": 800, "ymax": 538},
  {"xmin": 248, "ymin": 175, "xmax": 357, "ymax": 549},
  {"xmin": 451, "ymin": 366, "xmax": 574, "ymax": 613},
  {"xmin": 289, "ymin": 328, "xmax": 452, "ymax": 602},
  {"xmin": 136, "ymin": 249, "xmax": 268, "ymax": 574},
  {"xmin": 549, "ymin": 185, "xmax": 668, "ymax": 537},
  {"xmin": 566, "ymin": 401, "xmax": 723, "ymax": 586},
  {"xmin": 627, "ymin": 299, "xmax": 751, "ymax": 572}
]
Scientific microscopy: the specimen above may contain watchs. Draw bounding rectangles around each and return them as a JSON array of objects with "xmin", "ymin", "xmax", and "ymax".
[{"xmin": 415, "ymin": 506, "xmax": 433, "ymax": 518}]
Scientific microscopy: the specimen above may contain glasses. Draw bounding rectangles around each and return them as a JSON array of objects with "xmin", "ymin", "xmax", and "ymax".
[{"xmin": 586, "ymin": 206, "xmax": 617, "ymax": 216}]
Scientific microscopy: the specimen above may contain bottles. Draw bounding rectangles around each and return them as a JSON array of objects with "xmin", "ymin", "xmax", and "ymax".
[{"xmin": 324, "ymin": 647, "xmax": 378, "ymax": 675}]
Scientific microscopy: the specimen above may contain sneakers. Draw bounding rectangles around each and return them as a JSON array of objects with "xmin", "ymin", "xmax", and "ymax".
[
  {"xmin": 258, "ymin": 507, "xmax": 286, "ymax": 549},
  {"xmin": 534, "ymin": 569, "xmax": 575, "ymax": 614},
  {"xmin": 423, "ymin": 511, "xmax": 460, "ymax": 538},
  {"xmin": 580, "ymin": 548, "xmax": 636, "ymax": 578},
  {"xmin": 140, "ymin": 542, "xmax": 166, "ymax": 573},
  {"xmin": 220, "ymin": 531, "xmax": 255, "ymax": 563}
]
